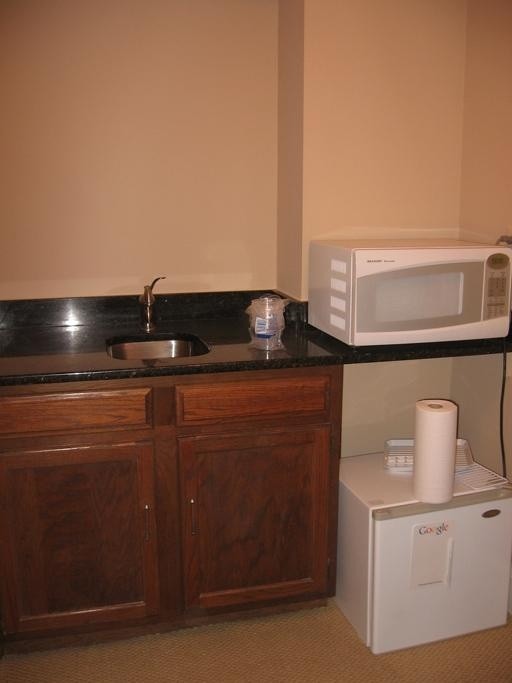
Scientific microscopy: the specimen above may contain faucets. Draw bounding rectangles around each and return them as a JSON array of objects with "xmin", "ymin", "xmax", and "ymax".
[{"xmin": 139, "ymin": 275, "xmax": 166, "ymax": 333}]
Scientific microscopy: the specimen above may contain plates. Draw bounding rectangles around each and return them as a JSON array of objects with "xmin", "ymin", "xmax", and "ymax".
[{"xmin": 383, "ymin": 435, "xmax": 474, "ymax": 474}]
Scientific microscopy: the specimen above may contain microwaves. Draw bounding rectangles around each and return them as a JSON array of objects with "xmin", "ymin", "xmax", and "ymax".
[{"xmin": 309, "ymin": 240, "xmax": 512, "ymax": 347}]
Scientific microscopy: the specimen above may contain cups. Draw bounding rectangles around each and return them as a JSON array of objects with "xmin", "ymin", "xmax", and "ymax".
[{"xmin": 250, "ymin": 297, "xmax": 283, "ymax": 350}]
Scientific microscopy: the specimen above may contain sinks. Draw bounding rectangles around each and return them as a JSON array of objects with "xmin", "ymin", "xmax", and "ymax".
[{"xmin": 104, "ymin": 331, "xmax": 212, "ymax": 360}]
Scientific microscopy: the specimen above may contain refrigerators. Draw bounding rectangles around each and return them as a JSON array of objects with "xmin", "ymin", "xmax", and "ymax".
[{"xmin": 334, "ymin": 451, "xmax": 512, "ymax": 656}]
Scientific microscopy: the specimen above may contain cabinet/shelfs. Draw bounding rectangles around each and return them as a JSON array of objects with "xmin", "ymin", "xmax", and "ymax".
[{"xmin": 1, "ymin": 366, "xmax": 342, "ymax": 652}]
